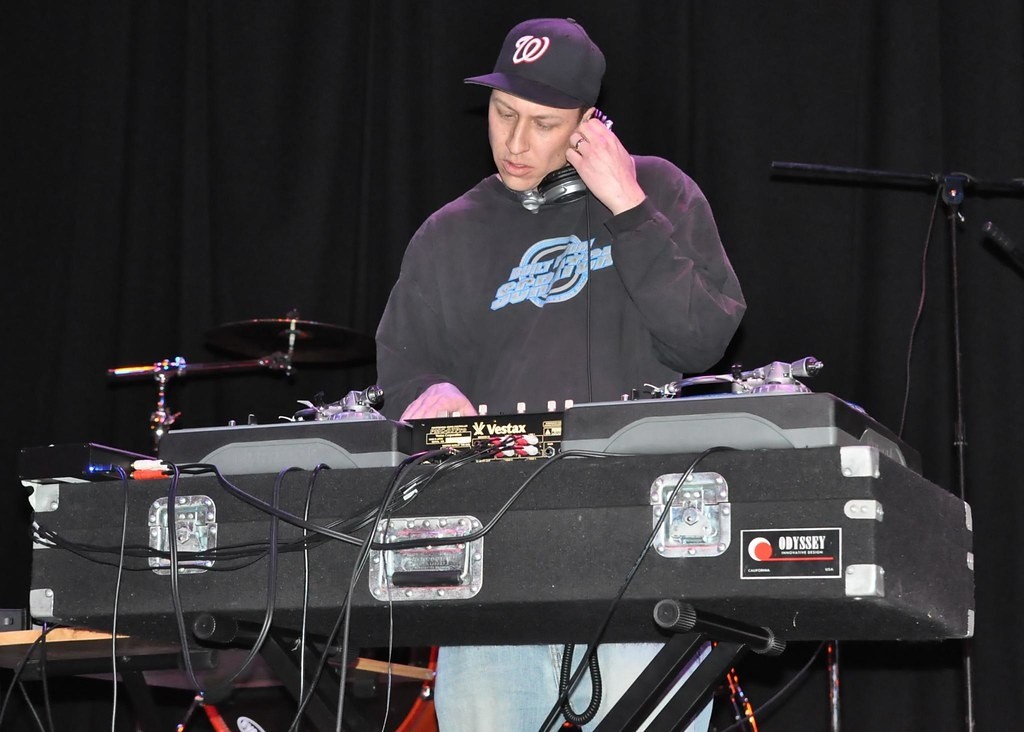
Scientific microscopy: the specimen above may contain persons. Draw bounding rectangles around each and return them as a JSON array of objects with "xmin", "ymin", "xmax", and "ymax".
[{"xmin": 377, "ymin": 17, "xmax": 746, "ymax": 732}]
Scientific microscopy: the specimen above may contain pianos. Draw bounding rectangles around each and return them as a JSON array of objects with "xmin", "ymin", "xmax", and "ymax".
[{"xmin": 153, "ymin": 393, "xmax": 928, "ymax": 485}]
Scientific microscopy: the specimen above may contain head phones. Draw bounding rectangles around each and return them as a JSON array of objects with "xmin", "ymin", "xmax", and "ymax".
[{"xmin": 519, "ymin": 110, "xmax": 613, "ymax": 210}]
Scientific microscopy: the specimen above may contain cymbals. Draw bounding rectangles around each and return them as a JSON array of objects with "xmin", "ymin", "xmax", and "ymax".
[{"xmin": 200, "ymin": 317, "xmax": 370, "ymax": 353}]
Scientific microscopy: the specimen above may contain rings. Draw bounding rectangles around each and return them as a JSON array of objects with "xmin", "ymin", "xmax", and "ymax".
[{"xmin": 575, "ymin": 138, "xmax": 585, "ymax": 151}]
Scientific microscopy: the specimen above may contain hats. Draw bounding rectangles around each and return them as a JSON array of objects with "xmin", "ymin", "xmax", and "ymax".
[{"xmin": 463, "ymin": 17, "xmax": 606, "ymax": 110}]
